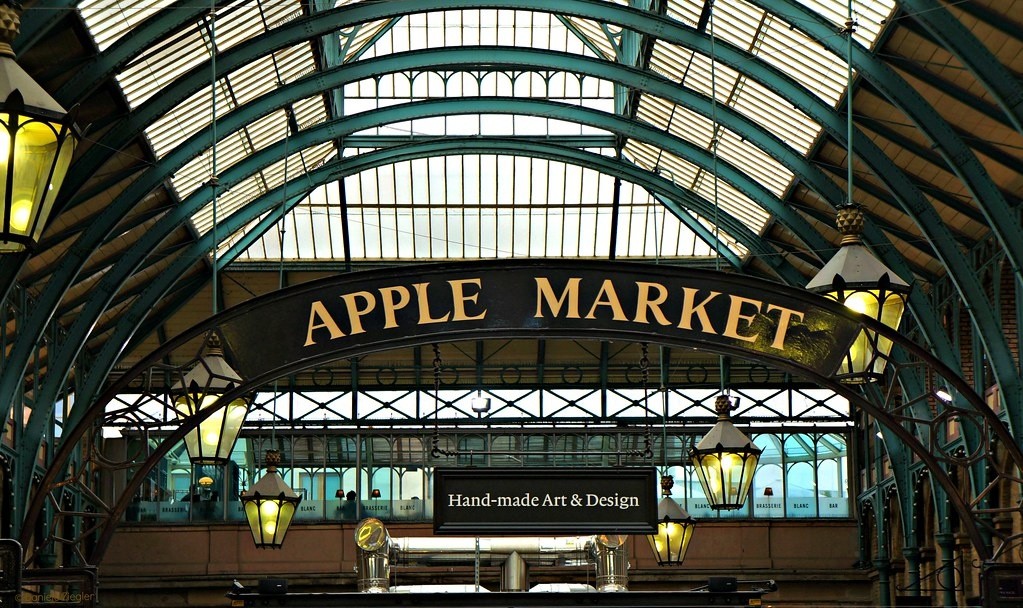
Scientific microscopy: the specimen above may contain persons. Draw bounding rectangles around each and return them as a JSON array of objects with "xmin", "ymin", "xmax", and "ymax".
[
  {"xmin": 406, "ymin": 497, "xmax": 422, "ymax": 519},
  {"xmin": 335, "ymin": 490, "xmax": 366, "ymax": 519},
  {"xmin": 181, "ymin": 485, "xmax": 199, "ymax": 514}
]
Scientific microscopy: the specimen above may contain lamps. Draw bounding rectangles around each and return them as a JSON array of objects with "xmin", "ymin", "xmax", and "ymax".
[
  {"xmin": 335, "ymin": 489, "xmax": 345, "ymax": 518},
  {"xmin": 936, "ymin": 386, "xmax": 953, "ymax": 404},
  {"xmin": 804, "ymin": 0, "xmax": 913, "ymax": 384},
  {"xmin": 239, "ymin": 106, "xmax": 304, "ymax": 550},
  {"xmin": 166, "ymin": 0, "xmax": 258, "ymax": 465},
  {"xmin": 764, "ymin": 487, "xmax": 774, "ymax": 516},
  {"xmin": 0, "ymin": 0, "xmax": 93, "ymax": 262},
  {"xmin": 371, "ymin": 489, "xmax": 382, "ymax": 516},
  {"xmin": 646, "ymin": 194, "xmax": 697, "ymax": 566},
  {"xmin": 684, "ymin": 0, "xmax": 767, "ymax": 511}
]
